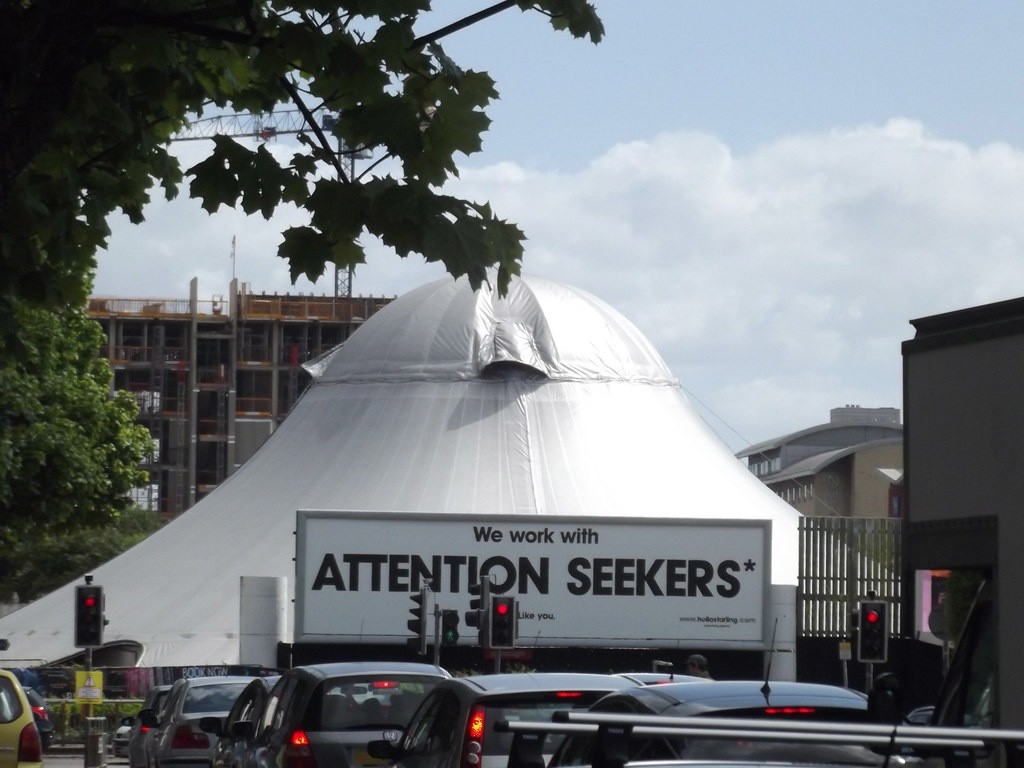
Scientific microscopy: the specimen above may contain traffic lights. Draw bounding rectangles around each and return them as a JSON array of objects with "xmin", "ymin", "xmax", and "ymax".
[
  {"xmin": 443, "ymin": 608, "xmax": 460, "ymax": 649},
  {"xmin": 406, "ymin": 593, "xmax": 422, "ymax": 654},
  {"xmin": 861, "ymin": 600, "xmax": 886, "ymax": 663},
  {"xmin": 465, "ymin": 583, "xmax": 483, "ymax": 630},
  {"xmin": 490, "ymin": 596, "xmax": 517, "ymax": 647},
  {"xmin": 73, "ymin": 586, "xmax": 102, "ymax": 647}
]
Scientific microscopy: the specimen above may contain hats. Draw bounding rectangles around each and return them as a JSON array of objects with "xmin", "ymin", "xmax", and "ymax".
[{"xmin": 684, "ymin": 654, "xmax": 707, "ymax": 666}]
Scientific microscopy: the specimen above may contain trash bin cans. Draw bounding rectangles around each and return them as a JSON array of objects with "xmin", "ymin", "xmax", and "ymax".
[{"xmin": 85, "ymin": 717, "xmax": 108, "ymax": 768}]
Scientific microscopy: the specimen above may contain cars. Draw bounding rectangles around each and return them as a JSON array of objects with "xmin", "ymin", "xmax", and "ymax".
[
  {"xmin": 543, "ymin": 680, "xmax": 926, "ymax": 768},
  {"xmin": 366, "ymin": 671, "xmax": 709, "ymax": 768},
  {"xmin": 229, "ymin": 662, "xmax": 461, "ymax": 768},
  {"xmin": 111, "ymin": 675, "xmax": 294, "ymax": 768},
  {"xmin": 21, "ymin": 685, "xmax": 56, "ymax": 755},
  {"xmin": 0, "ymin": 670, "xmax": 43, "ymax": 768}
]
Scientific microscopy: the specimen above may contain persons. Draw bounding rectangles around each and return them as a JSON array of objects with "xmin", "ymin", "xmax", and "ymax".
[{"xmin": 683, "ymin": 655, "xmax": 713, "ymax": 680}]
